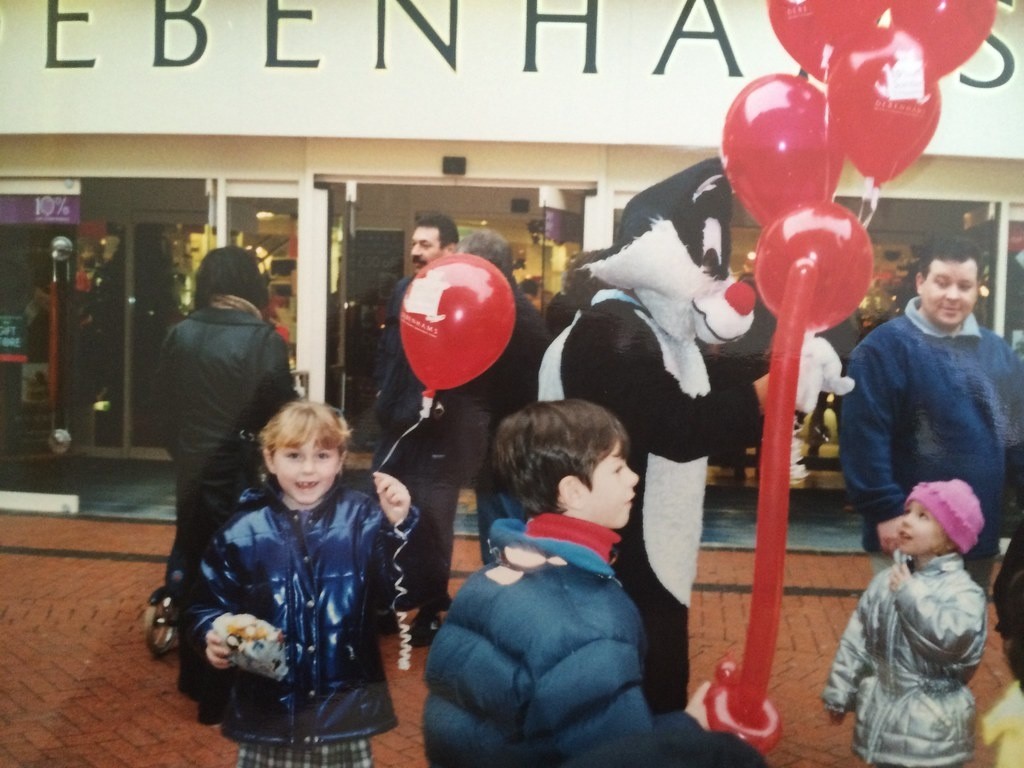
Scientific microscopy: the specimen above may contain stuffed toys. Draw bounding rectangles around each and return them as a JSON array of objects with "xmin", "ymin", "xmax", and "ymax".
[{"xmin": 536, "ymin": 157, "xmax": 858, "ymax": 707}]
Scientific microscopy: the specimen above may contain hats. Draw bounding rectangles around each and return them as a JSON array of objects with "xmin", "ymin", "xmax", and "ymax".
[{"xmin": 905, "ymin": 478, "xmax": 984, "ymax": 554}]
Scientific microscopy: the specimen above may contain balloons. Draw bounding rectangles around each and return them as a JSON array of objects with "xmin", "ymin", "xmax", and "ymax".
[
  {"xmin": 981, "ymin": 679, "xmax": 1024, "ymax": 768},
  {"xmin": 890, "ymin": 0, "xmax": 996, "ymax": 79},
  {"xmin": 701, "ymin": 202, "xmax": 874, "ymax": 747},
  {"xmin": 720, "ymin": 73, "xmax": 843, "ymax": 229},
  {"xmin": 766, "ymin": 0, "xmax": 891, "ymax": 83},
  {"xmin": 829, "ymin": 24, "xmax": 942, "ymax": 189}
]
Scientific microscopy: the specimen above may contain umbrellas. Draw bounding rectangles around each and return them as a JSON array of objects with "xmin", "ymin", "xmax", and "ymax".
[{"xmin": 401, "ymin": 254, "xmax": 517, "ymax": 416}]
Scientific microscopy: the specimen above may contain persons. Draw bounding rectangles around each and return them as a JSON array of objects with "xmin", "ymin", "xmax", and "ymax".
[
  {"xmin": 369, "ymin": 213, "xmax": 457, "ymax": 647},
  {"xmin": 423, "ymin": 395, "xmax": 770, "ymax": 768},
  {"xmin": 142, "ymin": 246, "xmax": 297, "ymax": 728},
  {"xmin": 459, "ymin": 223, "xmax": 545, "ymax": 573},
  {"xmin": 800, "ymin": 318, "xmax": 864, "ymax": 462},
  {"xmin": 518, "ymin": 276, "xmax": 576, "ymax": 341},
  {"xmin": 91, "ymin": 222, "xmax": 171, "ymax": 472},
  {"xmin": 837, "ymin": 234, "xmax": 1024, "ymax": 589},
  {"xmin": 175, "ymin": 399, "xmax": 438, "ymax": 768},
  {"xmin": 819, "ymin": 478, "xmax": 987, "ymax": 768},
  {"xmin": 991, "ymin": 521, "xmax": 1024, "ymax": 694},
  {"xmin": 718, "ymin": 271, "xmax": 772, "ymax": 415}
]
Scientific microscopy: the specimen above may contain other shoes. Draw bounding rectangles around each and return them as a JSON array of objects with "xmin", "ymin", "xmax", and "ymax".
[
  {"xmin": 376, "ymin": 612, "xmax": 399, "ymax": 634},
  {"xmin": 408, "ymin": 611, "xmax": 441, "ymax": 648}
]
